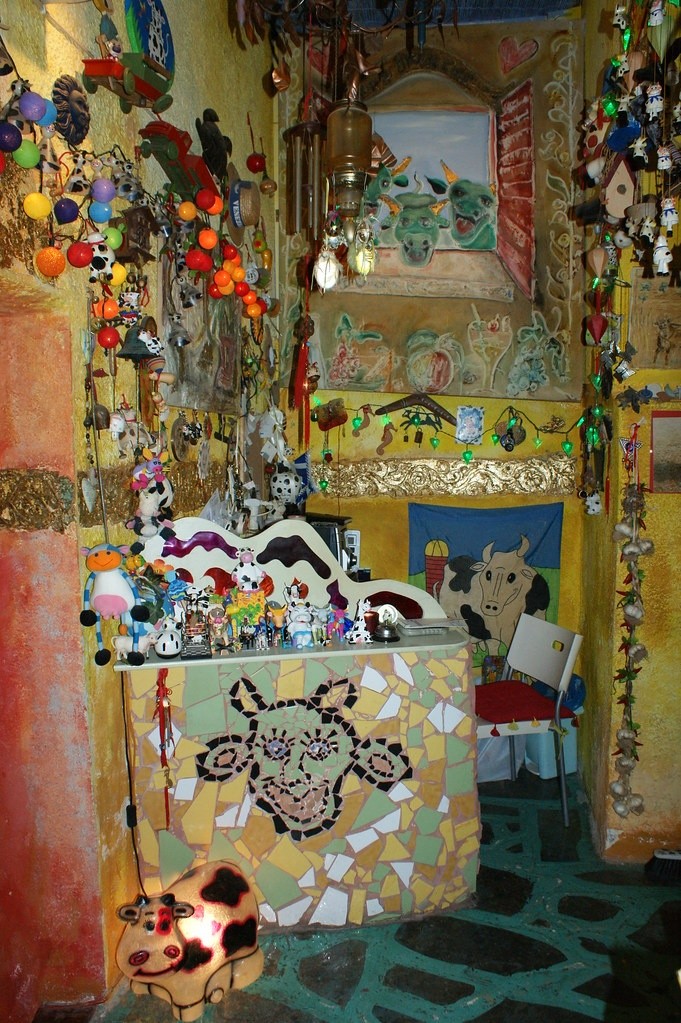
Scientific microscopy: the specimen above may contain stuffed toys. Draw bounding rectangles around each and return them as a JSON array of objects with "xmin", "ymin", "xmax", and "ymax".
[{"xmin": 77, "ymin": 544, "xmax": 150, "ymax": 667}]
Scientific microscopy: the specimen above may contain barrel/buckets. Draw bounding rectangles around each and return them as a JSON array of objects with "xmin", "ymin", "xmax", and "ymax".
[{"xmin": 524, "ymin": 705, "xmax": 585, "ymax": 780}]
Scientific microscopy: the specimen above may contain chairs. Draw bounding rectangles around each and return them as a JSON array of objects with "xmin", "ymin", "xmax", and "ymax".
[{"xmin": 468, "ymin": 612, "xmax": 587, "ymax": 829}]
